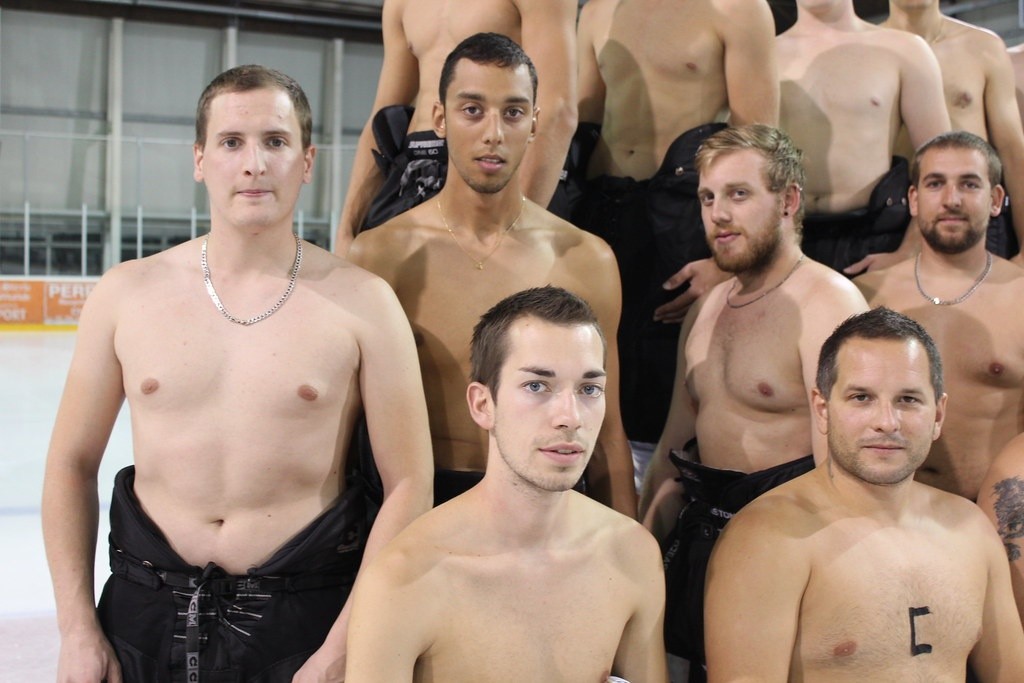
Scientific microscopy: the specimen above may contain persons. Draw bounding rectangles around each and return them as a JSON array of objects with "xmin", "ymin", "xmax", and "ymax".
[
  {"xmin": 638, "ymin": 120, "xmax": 874, "ymax": 683},
  {"xmin": 545, "ymin": 0, "xmax": 781, "ymax": 446},
  {"xmin": 847, "ymin": 130, "xmax": 1022, "ymax": 504},
  {"xmin": 984, "ymin": 43, "xmax": 1024, "ymax": 264},
  {"xmin": 876, "ymin": 0, "xmax": 1024, "ymax": 272},
  {"xmin": 38, "ymin": 62, "xmax": 437, "ymax": 683},
  {"xmin": 334, "ymin": 1, "xmax": 580, "ymax": 246},
  {"xmin": 724, "ymin": 0, "xmax": 952, "ymax": 282},
  {"xmin": 974, "ymin": 430, "xmax": 1024, "ymax": 621},
  {"xmin": 701, "ymin": 305, "xmax": 1024, "ymax": 683},
  {"xmin": 341, "ymin": 281, "xmax": 668, "ymax": 683}
]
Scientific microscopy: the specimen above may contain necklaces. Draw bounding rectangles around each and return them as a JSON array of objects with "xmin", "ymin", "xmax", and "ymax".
[
  {"xmin": 725, "ymin": 252, "xmax": 805, "ymax": 309},
  {"xmin": 437, "ymin": 193, "xmax": 527, "ymax": 270},
  {"xmin": 201, "ymin": 232, "xmax": 303, "ymax": 327},
  {"xmin": 914, "ymin": 249, "xmax": 992, "ymax": 305}
]
